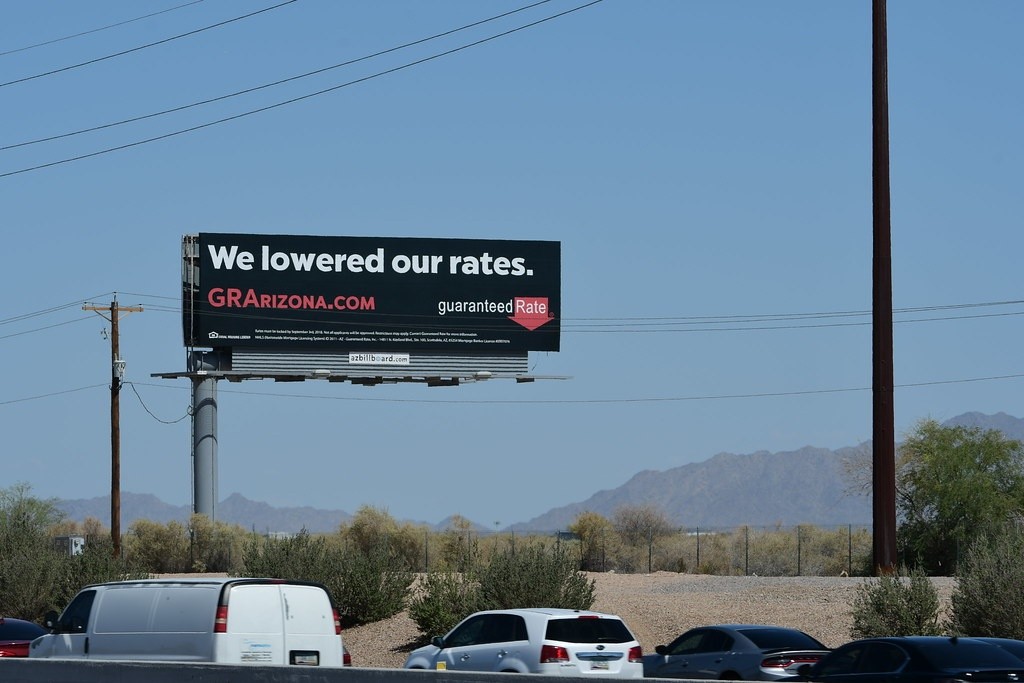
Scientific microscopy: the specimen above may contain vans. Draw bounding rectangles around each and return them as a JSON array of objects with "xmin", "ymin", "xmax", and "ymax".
[{"xmin": 26, "ymin": 579, "xmax": 354, "ymax": 669}]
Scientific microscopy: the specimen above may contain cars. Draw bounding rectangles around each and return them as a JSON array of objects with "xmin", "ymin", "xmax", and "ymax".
[
  {"xmin": 964, "ymin": 635, "xmax": 1024, "ymax": 665},
  {"xmin": 796, "ymin": 636, "xmax": 1024, "ymax": 683},
  {"xmin": 0, "ymin": 613, "xmax": 52, "ymax": 656},
  {"xmin": 403, "ymin": 606, "xmax": 644, "ymax": 679},
  {"xmin": 641, "ymin": 622, "xmax": 834, "ymax": 681}
]
